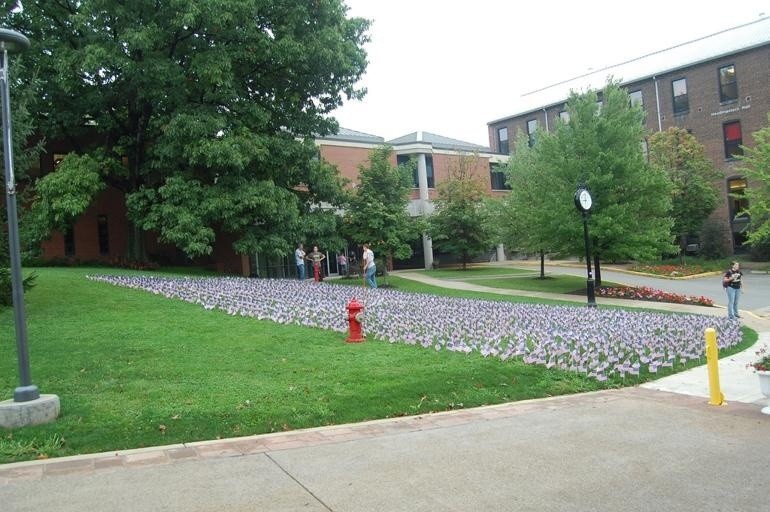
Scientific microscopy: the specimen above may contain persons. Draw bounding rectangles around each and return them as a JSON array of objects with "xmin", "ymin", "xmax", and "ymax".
[
  {"xmin": 722, "ymin": 261, "xmax": 744, "ymax": 320},
  {"xmin": 295, "ymin": 243, "xmax": 306, "ymax": 281},
  {"xmin": 362, "ymin": 243, "xmax": 378, "ymax": 288},
  {"xmin": 306, "ymin": 246, "xmax": 325, "ymax": 282},
  {"xmin": 338, "ymin": 252, "xmax": 346, "ymax": 274}
]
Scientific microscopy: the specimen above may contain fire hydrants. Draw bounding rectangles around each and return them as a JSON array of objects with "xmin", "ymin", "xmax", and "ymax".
[{"xmin": 342, "ymin": 295, "xmax": 368, "ymax": 343}]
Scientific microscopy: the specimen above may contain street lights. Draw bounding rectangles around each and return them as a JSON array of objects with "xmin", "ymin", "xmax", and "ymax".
[{"xmin": 0, "ymin": 28, "xmax": 64, "ymax": 431}]
